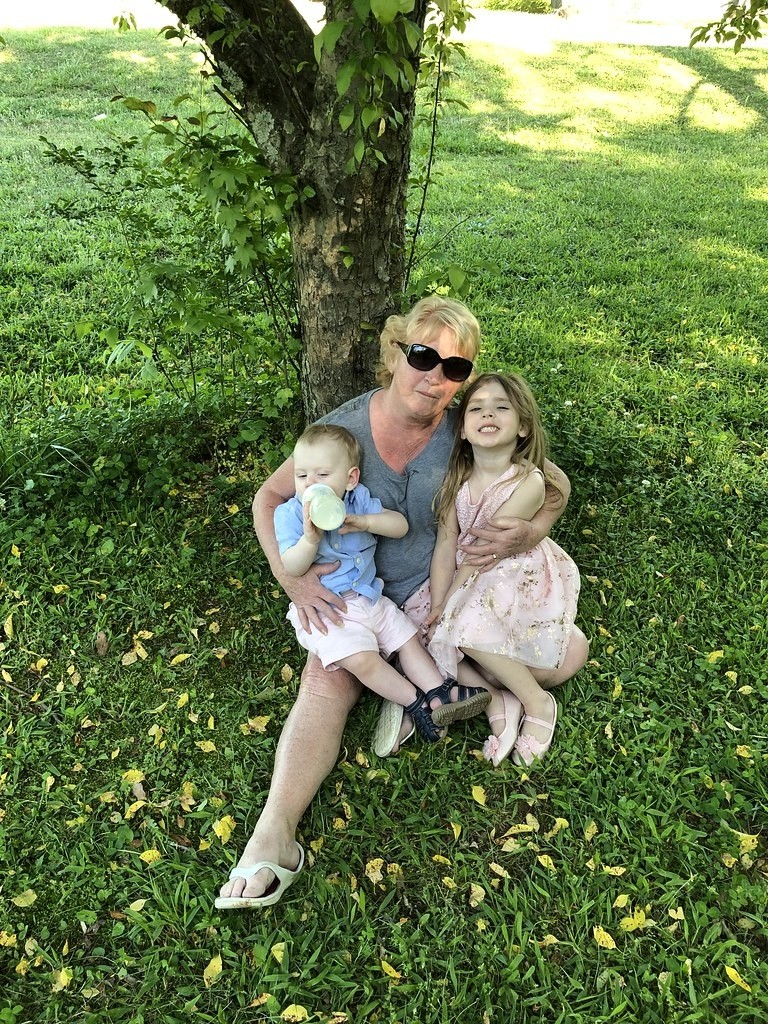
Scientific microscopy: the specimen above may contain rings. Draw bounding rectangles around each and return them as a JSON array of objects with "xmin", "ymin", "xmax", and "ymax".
[{"xmin": 493, "ymin": 553, "xmax": 498, "ymax": 559}]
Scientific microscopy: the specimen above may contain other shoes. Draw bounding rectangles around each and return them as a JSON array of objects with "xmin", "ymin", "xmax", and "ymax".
[
  {"xmin": 513, "ymin": 691, "xmax": 557, "ymax": 767},
  {"xmin": 483, "ymin": 690, "xmax": 524, "ymax": 767}
]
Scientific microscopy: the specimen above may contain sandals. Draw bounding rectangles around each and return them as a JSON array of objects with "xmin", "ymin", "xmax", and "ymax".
[{"xmin": 426, "ymin": 678, "xmax": 492, "ymax": 725}]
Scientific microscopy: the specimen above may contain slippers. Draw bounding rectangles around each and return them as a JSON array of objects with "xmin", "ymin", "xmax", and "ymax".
[
  {"xmin": 375, "ymin": 699, "xmax": 415, "ymax": 758},
  {"xmin": 212, "ymin": 841, "xmax": 304, "ymax": 910},
  {"xmin": 405, "ymin": 691, "xmax": 448, "ymax": 743}
]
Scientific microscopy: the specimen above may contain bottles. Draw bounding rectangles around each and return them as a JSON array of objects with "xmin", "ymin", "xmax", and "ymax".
[{"xmin": 301, "ymin": 483, "xmax": 348, "ymax": 531}]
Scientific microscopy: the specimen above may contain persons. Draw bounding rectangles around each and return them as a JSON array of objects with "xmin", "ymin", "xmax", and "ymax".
[
  {"xmin": 274, "ymin": 424, "xmax": 493, "ymax": 746},
  {"xmin": 213, "ymin": 293, "xmax": 590, "ymax": 908},
  {"xmin": 400, "ymin": 371, "xmax": 582, "ymax": 767}
]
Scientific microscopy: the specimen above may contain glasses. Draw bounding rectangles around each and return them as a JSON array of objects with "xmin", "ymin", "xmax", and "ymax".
[{"xmin": 397, "ymin": 341, "xmax": 474, "ymax": 382}]
